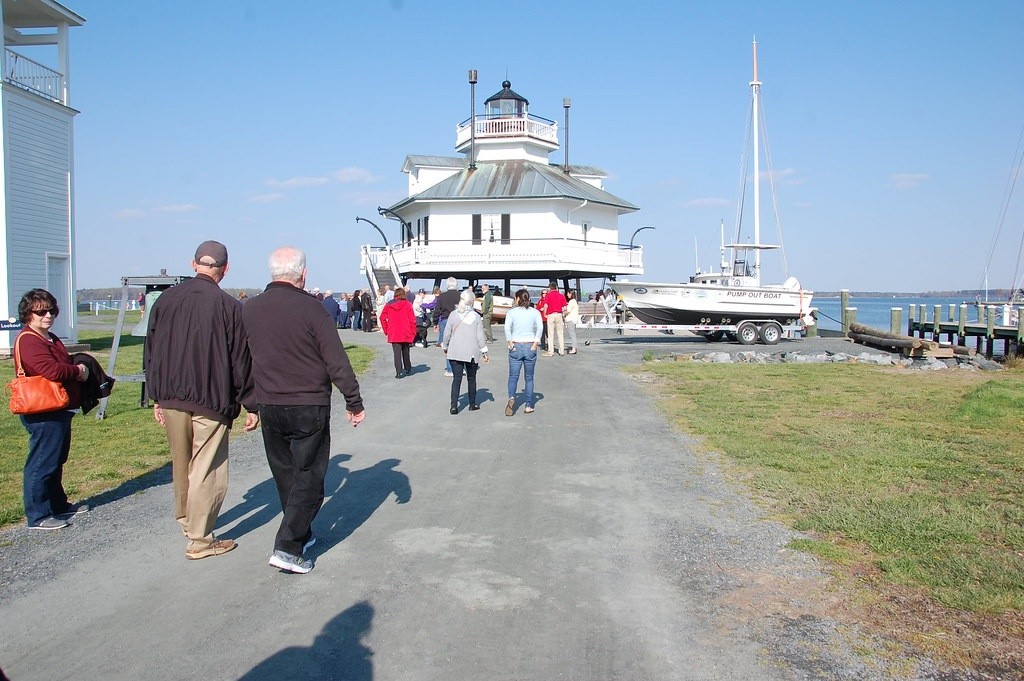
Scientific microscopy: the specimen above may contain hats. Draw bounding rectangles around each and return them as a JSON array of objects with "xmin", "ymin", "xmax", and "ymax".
[
  {"xmin": 195, "ymin": 240, "xmax": 228, "ymax": 267},
  {"xmin": 419, "ymin": 289, "xmax": 426, "ymax": 293}
]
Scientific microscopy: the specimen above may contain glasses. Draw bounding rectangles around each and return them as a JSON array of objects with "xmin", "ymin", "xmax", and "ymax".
[
  {"xmin": 542, "ymin": 292, "xmax": 547, "ymax": 295},
  {"xmin": 29, "ymin": 308, "xmax": 55, "ymax": 316}
]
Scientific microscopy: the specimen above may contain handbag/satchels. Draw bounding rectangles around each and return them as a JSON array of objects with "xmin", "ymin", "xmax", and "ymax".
[{"xmin": 5, "ymin": 331, "xmax": 69, "ymax": 414}]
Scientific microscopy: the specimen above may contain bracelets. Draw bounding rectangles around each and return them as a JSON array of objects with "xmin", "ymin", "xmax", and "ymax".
[{"xmin": 511, "ymin": 346, "xmax": 514, "ymax": 351}]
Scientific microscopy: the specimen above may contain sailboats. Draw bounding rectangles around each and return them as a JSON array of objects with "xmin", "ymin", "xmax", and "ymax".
[
  {"xmin": 959, "ymin": 118, "xmax": 1024, "ymax": 330},
  {"xmin": 603, "ymin": 33, "xmax": 815, "ymax": 326}
]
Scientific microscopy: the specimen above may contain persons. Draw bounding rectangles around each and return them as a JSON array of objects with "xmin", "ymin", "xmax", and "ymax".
[
  {"xmin": 504, "ymin": 290, "xmax": 543, "ymax": 416},
  {"xmin": 442, "ymin": 289, "xmax": 489, "ymax": 414},
  {"xmin": 242, "ymin": 247, "xmax": 365, "ymax": 573},
  {"xmin": 433, "ymin": 277, "xmax": 472, "ymax": 376},
  {"xmin": 475, "ymin": 284, "xmax": 493, "ymax": 343},
  {"xmin": 544, "ymin": 282, "xmax": 566, "ymax": 356},
  {"xmin": 587, "ymin": 288, "xmax": 616, "ymax": 302},
  {"xmin": 404, "ymin": 286, "xmax": 416, "ymax": 304},
  {"xmin": 306, "ymin": 287, "xmax": 374, "ymax": 332},
  {"xmin": 537, "ymin": 289, "xmax": 548, "ymax": 350},
  {"xmin": 420, "ymin": 288, "xmax": 441, "ymax": 348},
  {"xmin": 143, "ymin": 240, "xmax": 259, "ymax": 559},
  {"xmin": 412, "ymin": 289, "xmax": 426, "ymax": 346},
  {"xmin": 375, "ymin": 284, "xmax": 399, "ymax": 332},
  {"xmin": 564, "ymin": 289, "xmax": 579, "ymax": 354},
  {"xmin": 138, "ymin": 292, "xmax": 145, "ymax": 318},
  {"xmin": 14, "ymin": 289, "xmax": 89, "ymax": 529},
  {"xmin": 512, "ymin": 286, "xmax": 527, "ymax": 307},
  {"xmin": 379, "ymin": 288, "xmax": 417, "ymax": 378}
]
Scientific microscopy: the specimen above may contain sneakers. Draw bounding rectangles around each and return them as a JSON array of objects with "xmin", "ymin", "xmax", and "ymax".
[
  {"xmin": 301, "ymin": 531, "xmax": 316, "ymax": 554},
  {"xmin": 55, "ymin": 504, "xmax": 90, "ymax": 515},
  {"xmin": 268, "ymin": 550, "xmax": 313, "ymax": 574},
  {"xmin": 187, "ymin": 539, "xmax": 235, "ymax": 560},
  {"xmin": 27, "ymin": 517, "xmax": 68, "ymax": 529}
]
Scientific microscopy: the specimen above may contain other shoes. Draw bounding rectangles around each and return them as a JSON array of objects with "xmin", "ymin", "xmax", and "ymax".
[
  {"xmin": 394, "ymin": 372, "xmax": 404, "ymax": 378},
  {"xmin": 541, "ymin": 354, "xmax": 553, "ymax": 357},
  {"xmin": 486, "ymin": 339, "xmax": 493, "ymax": 343},
  {"xmin": 524, "ymin": 406, "xmax": 534, "ymax": 413},
  {"xmin": 505, "ymin": 398, "xmax": 515, "ymax": 416},
  {"xmin": 450, "ymin": 407, "xmax": 458, "ymax": 414},
  {"xmin": 444, "ymin": 371, "xmax": 454, "ymax": 376},
  {"xmin": 469, "ymin": 404, "xmax": 480, "ymax": 411},
  {"xmin": 568, "ymin": 349, "xmax": 577, "ymax": 354},
  {"xmin": 406, "ymin": 370, "xmax": 412, "ymax": 376}
]
